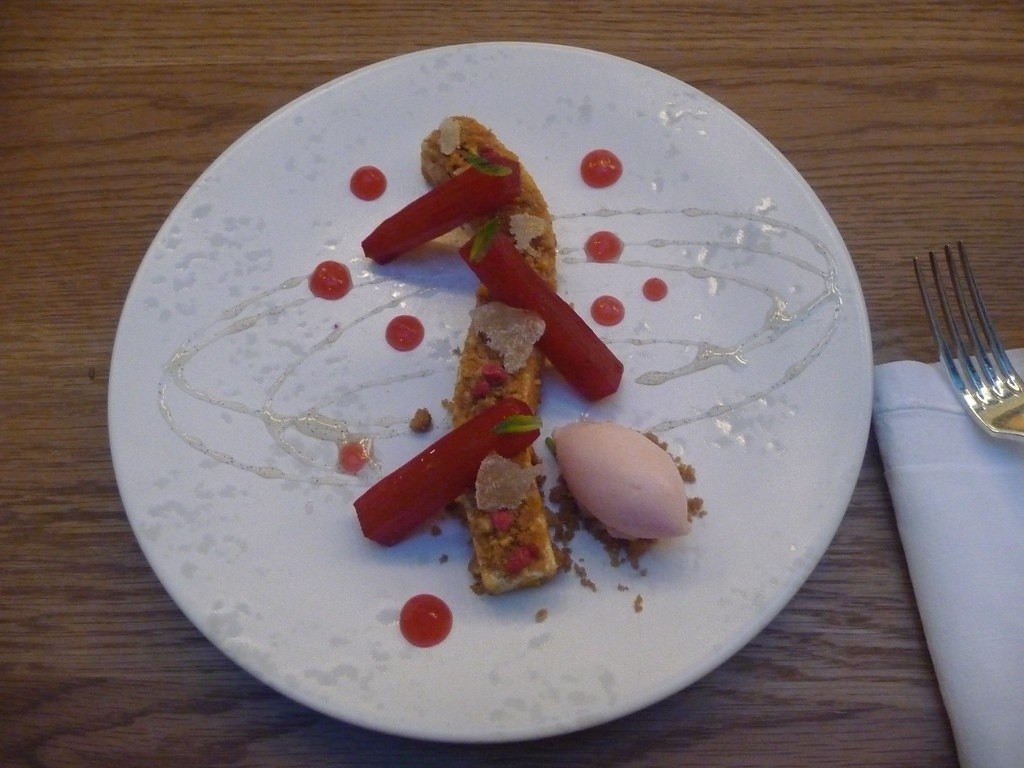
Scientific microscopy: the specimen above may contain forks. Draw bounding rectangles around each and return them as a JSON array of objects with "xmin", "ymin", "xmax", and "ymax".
[{"xmin": 912, "ymin": 239, "xmax": 1024, "ymax": 443}]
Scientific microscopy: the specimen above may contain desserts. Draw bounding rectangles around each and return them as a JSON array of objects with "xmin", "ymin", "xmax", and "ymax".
[
  {"xmin": 357, "ymin": 111, "xmax": 627, "ymax": 597},
  {"xmin": 548, "ymin": 420, "xmax": 691, "ymax": 539}
]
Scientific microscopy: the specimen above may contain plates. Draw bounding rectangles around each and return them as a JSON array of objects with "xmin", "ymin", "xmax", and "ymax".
[{"xmin": 107, "ymin": 40, "xmax": 876, "ymax": 746}]
